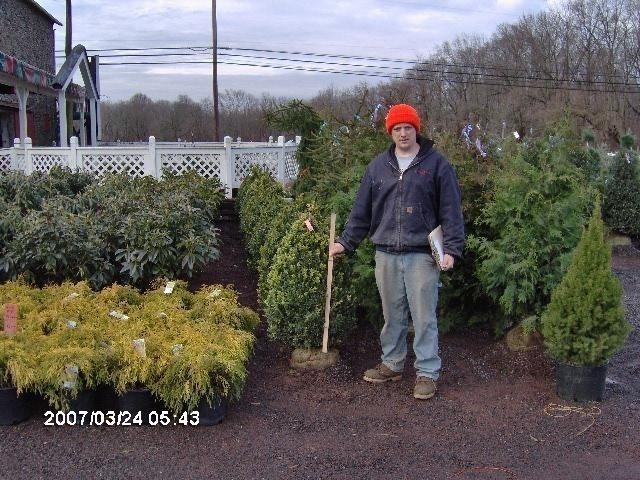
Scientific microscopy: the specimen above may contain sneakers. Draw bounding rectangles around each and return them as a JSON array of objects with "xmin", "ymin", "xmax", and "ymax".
[
  {"xmin": 363, "ymin": 363, "xmax": 403, "ymax": 384},
  {"xmin": 413, "ymin": 375, "xmax": 438, "ymax": 400}
]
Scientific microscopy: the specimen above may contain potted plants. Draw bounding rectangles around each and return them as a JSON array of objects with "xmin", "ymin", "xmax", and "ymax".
[
  {"xmin": 0, "ymin": 302, "xmax": 46, "ymax": 426},
  {"xmin": 108, "ymin": 326, "xmax": 167, "ymax": 422},
  {"xmin": 168, "ymin": 326, "xmax": 254, "ymax": 428},
  {"xmin": 46, "ymin": 324, "xmax": 108, "ymax": 418},
  {"xmin": 538, "ymin": 189, "xmax": 640, "ymax": 405}
]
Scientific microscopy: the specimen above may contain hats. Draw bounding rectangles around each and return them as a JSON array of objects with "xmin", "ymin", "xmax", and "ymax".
[{"xmin": 385, "ymin": 104, "xmax": 421, "ymax": 137}]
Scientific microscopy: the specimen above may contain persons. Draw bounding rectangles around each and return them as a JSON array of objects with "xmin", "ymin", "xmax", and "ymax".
[{"xmin": 329, "ymin": 103, "xmax": 465, "ymax": 400}]
